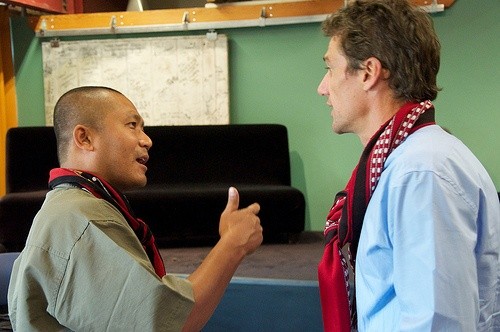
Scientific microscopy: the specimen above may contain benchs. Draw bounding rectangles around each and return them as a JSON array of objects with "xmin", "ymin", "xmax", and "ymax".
[{"xmin": 0, "ymin": 124, "xmax": 305, "ymax": 252}]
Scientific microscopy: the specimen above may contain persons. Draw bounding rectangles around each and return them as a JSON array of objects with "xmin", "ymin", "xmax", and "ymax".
[
  {"xmin": 6, "ymin": 85, "xmax": 265, "ymax": 332},
  {"xmin": 304, "ymin": 0, "xmax": 499, "ymax": 332}
]
